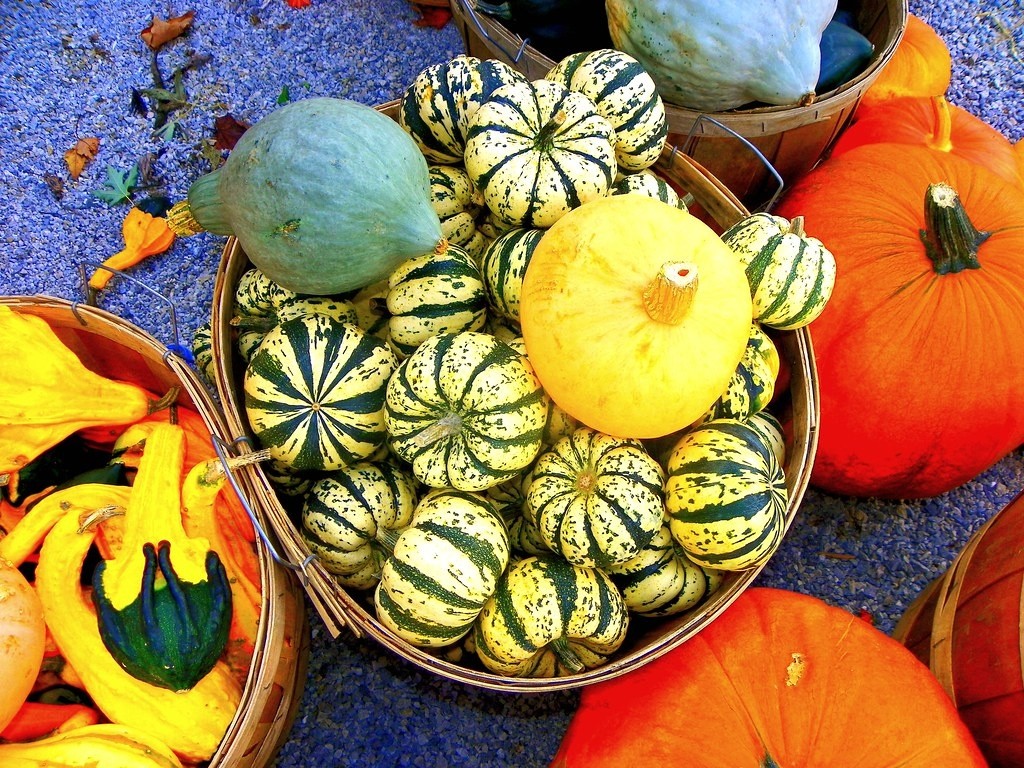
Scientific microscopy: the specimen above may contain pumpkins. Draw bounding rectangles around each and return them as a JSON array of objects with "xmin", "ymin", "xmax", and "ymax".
[{"xmin": 0, "ymin": 0, "xmax": 1024, "ymax": 768}]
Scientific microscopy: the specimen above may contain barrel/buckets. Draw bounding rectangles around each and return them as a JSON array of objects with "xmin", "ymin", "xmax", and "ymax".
[
  {"xmin": 889, "ymin": 490, "xmax": 1024, "ymax": 768},
  {"xmin": 0, "ymin": 260, "xmax": 307, "ymax": 768},
  {"xmin": 209, "ymin": 96, "xmax": 822, "ymax": 696},
  {"xmin": 450, "ymin": 0, "xmax": 908, "ymax": 213}
]
